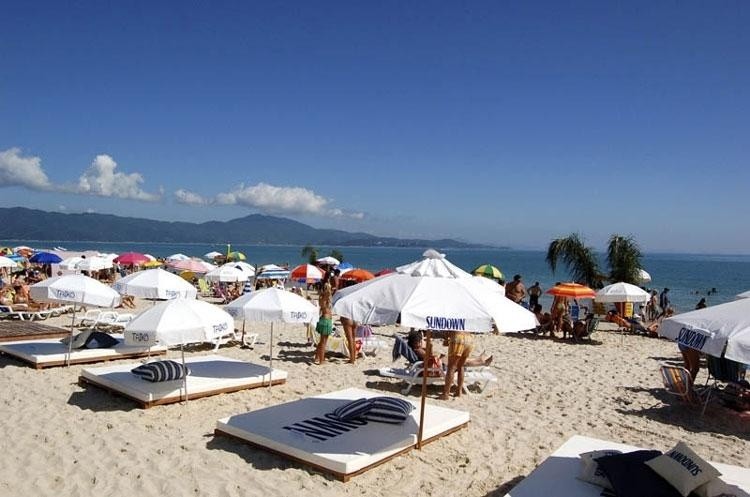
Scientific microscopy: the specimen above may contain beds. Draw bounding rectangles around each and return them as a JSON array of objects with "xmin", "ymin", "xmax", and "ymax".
[
  {"xmin": 0, "ymin": 337, "xmax": 167, "ymax": 370},
  {"xmin": 79, "ymin": 355, "xmax": 288, "ymax": 409},
  {"xmin": 214, "ymin": 386, "xmax": 471, "ymax": 482}
]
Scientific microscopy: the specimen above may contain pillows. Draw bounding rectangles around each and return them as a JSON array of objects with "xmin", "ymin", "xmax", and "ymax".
[
  {"xmin": 86, "ymin": 333, "xmax": 121, "ymax": 349},
  {"xmin": 333, "ymin": 398, "xmax": 376, "ymax": 421},
  {"xmin": 71, "ymin": 329, "xmax": 92, "ymax": 349},
  {"xmin": 579, "ymin": 449, "xmax": 623, "ymax": 465},
  {"xmin": 358, "ymin": 397, "xmax": 416, "ymax": 424},
  {"xmin": 694, "ymin": 478, "xmax": 732, "ymax": 496},
  {"xmin": 594, "ymin": 449, "xmax": 683, "ymax": 496},
  {"xmin": 644, "ymin": 441, "xmax": 724, "ymax": 496},
  {"xmin": 577, "ymin": 452, "xmax": 622, "ymax": 492},
  {"xmin": 60, "ymin": 336, "xmax": 72, "ymax": 344},
  {"xmin": 132, "ymin": 361, "xmax": 192, "ymax": 382}
]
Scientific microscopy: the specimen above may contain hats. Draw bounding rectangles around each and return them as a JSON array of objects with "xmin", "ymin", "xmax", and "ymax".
[{"xmin": 633, "ymin": 313, "xmax": 641, "ymax": 318}]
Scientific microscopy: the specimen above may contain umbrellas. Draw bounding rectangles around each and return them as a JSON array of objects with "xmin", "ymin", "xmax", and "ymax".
[
  {"xmin": 659, "ymin": 297, "xmax": 750, "ymax": 395},
  {"xmin": 166, "ymin": 257, "xmax": 217, "ymax": 273},
  {"xmin": 222, "ymin": 287, "xmax": 319, "ymax": 391},
  {"xmin": 114, "ymin": 268, "xmax": 198, "ymax": 307},
  {"xmin": 736, "ymin": 291, "xmax": 750, "ymax": 300},
  {"xmin": 30, "ymin": 272, "xmax": 122, "ymax": 369},
  {"xmin": 638, "ymin": 269, "xmax": 651, "ymax": 282},
  {"xmin": 332, "ymin": 248, "xmax": 541, "ymax": 452},
  {"xmin": 291, "ymin": 256, "xmax": 397, "ymax": 290},
  {"xmin": 124, "ymin": 298, "xmax": 235, "ymax": 404},
  {"xmin": 470, "ymin": 264, "xmax": 503, "ymax": 280},
  {"xmin": 203, "ymin": 249, "xmax": 291, "ymax": 282},
  {"xmin": 594, "ymin": 281, "xmax": 651, "ymax": 343},
  {"xmin": 545, "ymin": 281, "xmax": 596, "ymax": 338},
  {"xmin": 0, "ymin": 246, "xmax": 164, "ymax": 284},
  {"xmin": 168, "ymin": 253, "xmax": 191, "ymax": 261}
]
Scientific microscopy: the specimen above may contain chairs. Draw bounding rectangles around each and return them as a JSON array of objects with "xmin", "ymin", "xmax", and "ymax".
[
  {"xmin": 67, "ymin": 310, "xmax": 102, "ymax": 327},
  {"xmin": 0, "ymin": 305, "xmax": 52, "ymax": 322},
  {"xmin": 102, "ymin": 314, "xmax": 136, "ymax": 331},
  {"xmin": 381, "ymin": 361, "xmax": 497, "ymax": 396},
  {"xmin": 658, "ymin": 363, "xmax": 726, "ymax": 431},
  {"xmin": 570, "ymin": 318, "xmax": 600, "ymax": 344},
  {"xmin": 85, "ymin": 312, "xmax": 118, "ymax": 328},
  {"xmin": 204, "ymin": 331, "xmax": 258, "ymax": 353},
  {"xmin": 392, "ymin": 332, "xmax": 491, "ymax": 372},
  {"xmin": 307, "ymin": 322, "xmax": 389, "ymax": 360}
]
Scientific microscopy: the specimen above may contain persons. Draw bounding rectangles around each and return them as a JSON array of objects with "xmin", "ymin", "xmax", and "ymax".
[
  {"xmin": 695, "ymin": 298, "xmax": 707, "ymax": 310},
  {"xmin": 341, "ymin": 281, "xmax": 361, "ymax": 363},
  {"xmin": 679, "ymin": 343, "xmax": 700, "ymax": 383},
  {"xmin": 0, "ymin": 264, "xmax": 60, "ymax": 312},
  {"xmin": 211, "ymin": 281, "xmax": 243, "ymax": 305},
  {"xmin": 551, "ymin": 282, "xmax": 568, "ymax": 333},
  {"xmin": 435, "ymin": 331, "xmax": 473, "ymax": 401},
  {"xmin": 498, "ymin": 280, "xmax": 506, "ymax": 288},
  {"xmin": 408, "ymin": 331, "xmax": 493, "ymax": 369},
  {"xmin": 608, "ymin": 310, "xmax": 658, "ymax": 332},
  {"xmin": 527, "ymin": 282, "xmax": 542, "ymax": 310},
  {"xmin": 638, "ymin": 286, "xmax": 673, "ymax": 322},
  {"xmin": 315, "ymin": 283, "xmax": 332, "ymax": 365},
  {"xmin": 533, "ymin": 304, "xmax": 555, "ymax": 338},
  {"xmin": 695, "ymin": 287, "xmax": 719, "ymax": 297},
  {"xmin": 506, "ymin": 275, "xmax": 526, "ymax": 304},
  {"xmin": 99, "ymin": 270, "xmax": 137, "ymax": 310}
]
